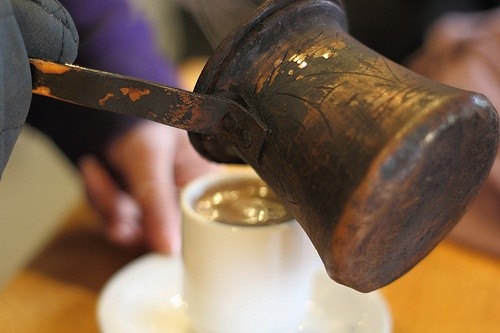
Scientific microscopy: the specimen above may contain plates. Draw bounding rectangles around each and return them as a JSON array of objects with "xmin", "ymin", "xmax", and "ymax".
[{"xmin": 94, "ymin": 245, "xmax": 392, "ymax": 333}]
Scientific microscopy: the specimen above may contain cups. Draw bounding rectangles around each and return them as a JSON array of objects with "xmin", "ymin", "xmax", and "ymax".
[
  {"xmin": 179, "ymin": 169, "xmax": 312, "ymax": 332},
  {"xmin": 28, "ymin": 1, "xmax": 499, "ymax": 292}
]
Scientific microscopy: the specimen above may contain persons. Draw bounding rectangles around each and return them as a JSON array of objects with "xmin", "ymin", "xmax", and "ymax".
[
  {"xmin": 2, "ymin": 1, "xmax": 232, "ymax": 257},
  {"xmin": 281, "ymin": 1, "xmax": 500, "ymax": 196}
]
